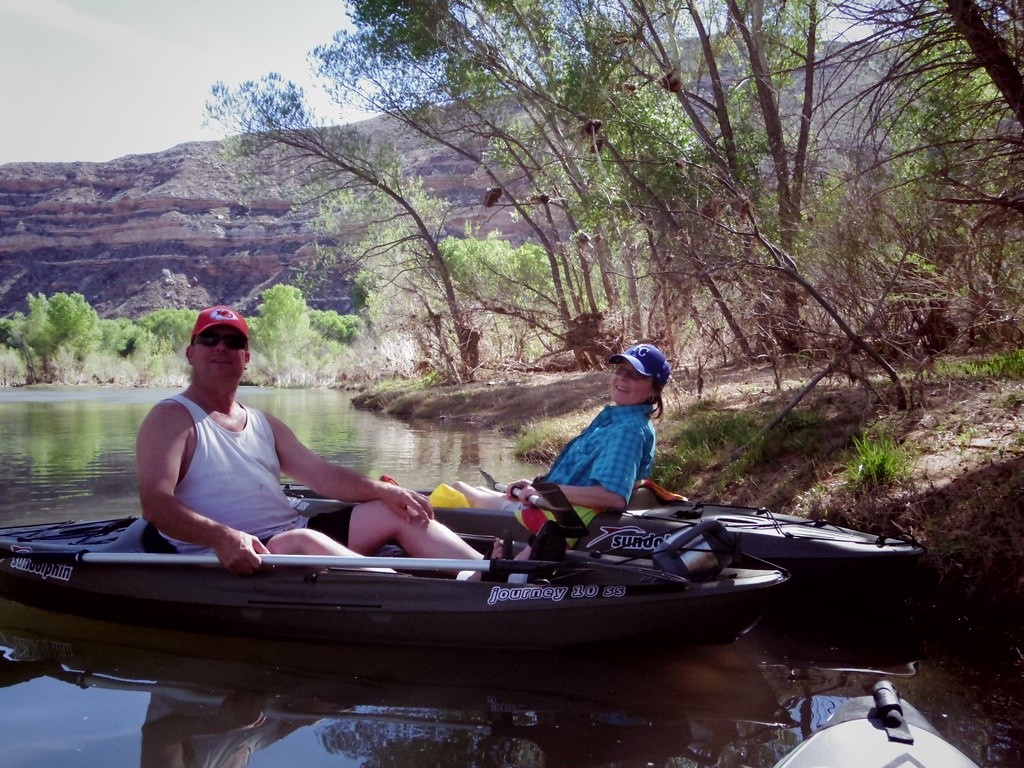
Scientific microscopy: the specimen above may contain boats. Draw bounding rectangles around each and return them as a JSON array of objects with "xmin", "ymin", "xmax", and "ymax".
[{"xmin": 0, "ymin": 468, "xmax": 924, "ymax": 650}]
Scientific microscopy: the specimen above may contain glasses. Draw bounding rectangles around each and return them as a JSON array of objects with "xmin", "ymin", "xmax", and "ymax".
[
  {"xmin": 193, "ymin": 330, "xmax": 248, "ymax": 351},
  {"xmin": 613, "ymin": 365, "xmax": 650, "ymax": 381}
]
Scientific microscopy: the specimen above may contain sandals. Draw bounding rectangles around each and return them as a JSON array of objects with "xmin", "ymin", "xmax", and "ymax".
[
  {"xmin": 480, "ymin": 528, "xmax": 513, "ymax": 582},
  {"xmin": 511, "ymin": 520, "xmax": 567, "ymax": 583}
]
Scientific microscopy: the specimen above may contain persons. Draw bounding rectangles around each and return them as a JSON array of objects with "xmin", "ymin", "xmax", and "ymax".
[
  {"xmin": 135, "ymin": 305, "xmax": 567, "ymax": 585},
  {"xmin": 451, "ymin": 342, "xmax": 670, "ymax": 512}
]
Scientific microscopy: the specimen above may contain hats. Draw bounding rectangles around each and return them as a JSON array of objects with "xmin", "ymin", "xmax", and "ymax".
[
  {"xmin": 192, "ymin": 306, "xmax": 249, "ymax": 347},
  {"xmin": 608, "ymin": 344, "xmax": 671, "ymax": 385}
]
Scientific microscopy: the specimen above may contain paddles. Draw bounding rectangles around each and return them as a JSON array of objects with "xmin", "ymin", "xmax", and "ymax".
[
  {"xmin": 478, "ymin": 466, "xmax": 590, "ymax": 538},
  {"xmin": 1, "ymin": 651, "xmax": 693, "ymax": 762},
  {"xmin": 0, "ymin": 545, "xmax": 692, "ymax": 585}
]
